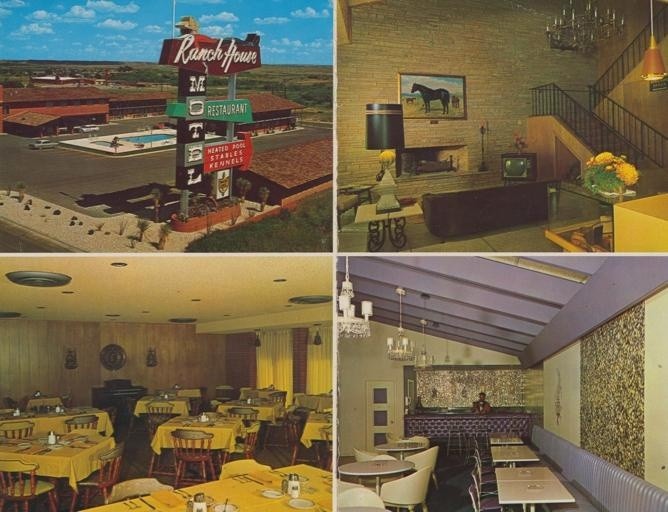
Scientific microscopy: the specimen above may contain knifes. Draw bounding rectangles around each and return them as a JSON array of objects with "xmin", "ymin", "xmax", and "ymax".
[
  {"xmin": 243, "ymin": 475, "xmax": 265, "ymax": 486},
  {"xmin": 137, "ymin": 495, "xmax": 158, "ymax": 510}
]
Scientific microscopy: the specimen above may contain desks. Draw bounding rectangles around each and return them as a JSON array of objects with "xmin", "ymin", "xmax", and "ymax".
[
  {"xmin": 352, "ymin": 197, "xmax": 426, "ymax": 252},
  {"xmin": 343, "ymin": 183, "xmax": 379, "ymax": 203},
  {"xmin": 543, "ymin": 214, "xmax": 614, "ymax": 252}
]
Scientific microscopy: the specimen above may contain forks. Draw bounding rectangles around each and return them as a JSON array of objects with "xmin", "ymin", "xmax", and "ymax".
[
  {"xmin": 174, "ymin": 488, "xmax": 193, "ymax": 499},
  {"xmin": 124, "ymin": 500, "xmax": 141, "ymax": 511}
]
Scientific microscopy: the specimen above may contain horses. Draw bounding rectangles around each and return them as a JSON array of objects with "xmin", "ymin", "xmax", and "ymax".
[
  {"xmin": 411, "ymin": 82, "xmax": 451, "ymax": 115},
  {"xmin": 406, "ymin": 97, "xmax": 416, "ymax": 103},
  {"xmin": 452, "ymin": 96, "xmax": 459, "ymax": 109}
]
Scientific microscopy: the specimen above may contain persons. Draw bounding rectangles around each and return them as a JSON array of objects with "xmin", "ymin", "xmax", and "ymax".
[{"xmin": 472, "ymin": 394, "xmax": 491, "ymax": 415}]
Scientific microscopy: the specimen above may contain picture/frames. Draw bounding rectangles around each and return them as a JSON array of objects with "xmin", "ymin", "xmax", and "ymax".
[
  {"xmin": 397, "ymin": 72, "xmax": 468, "ymax": 121},
  {"xmin": 648, "ymin": 77, "xmax": 668, "ymax": 92}
]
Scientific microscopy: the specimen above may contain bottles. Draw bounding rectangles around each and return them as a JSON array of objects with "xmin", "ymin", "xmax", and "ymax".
[
  {"xmin": 13, "ymin": 390, "xmax": 66, "ymax": 419},
  {"xmin": 48, "ymin": 428, "xmax": 56, "ymax": 445},
  {"xmin": 287, "ymin": 472, "xmax": 302, "ymax": 497},
  {"xmin": 175, "ymin": 384, "xmax": 178, "ymax": 389},
  {"xmin": 200, "ymin": 411, "xmax": 209, "ymax": 423},
  {"xmin": 184, "ymin": 499, "xmax": 193, "ymax": 511},
  {"xmin": 247, "ymin": 396, "xmax": 252, "ymax": 404},
  {"xmin": 164, "ymin": 393, "xmax": 169, "ymax": 399},
  {"xmin": 193, "ymin": 493, "xmax": 207, "ymax": 512}
]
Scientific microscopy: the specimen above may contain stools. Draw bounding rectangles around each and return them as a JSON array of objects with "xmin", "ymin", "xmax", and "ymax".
[{"xmin": 337, "ymin": 186, "xmax": 359, "ymax": 232}]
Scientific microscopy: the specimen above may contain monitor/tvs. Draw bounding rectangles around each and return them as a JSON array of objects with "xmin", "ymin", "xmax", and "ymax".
[{"xmin": 500, "ymin": 153, "xmax": 538, "ymax": 181}]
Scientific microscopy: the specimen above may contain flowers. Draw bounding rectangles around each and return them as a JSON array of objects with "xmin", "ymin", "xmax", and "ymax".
[
  {"xmin": 507, "ymin": 133, "xmax": 527, "ymax": 145},
  {"xmin": 572, "ymin": 151, "xmax": 640, "ymax": 195}
]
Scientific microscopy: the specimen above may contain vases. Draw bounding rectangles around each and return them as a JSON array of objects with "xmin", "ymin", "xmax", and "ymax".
[
  {"xmin": 515, "ymin": 147, "xmax": 522, "ymax": 155},
  {"xmin": 605, "ymin": 184, "xmax": 626, "ymax": 193}
]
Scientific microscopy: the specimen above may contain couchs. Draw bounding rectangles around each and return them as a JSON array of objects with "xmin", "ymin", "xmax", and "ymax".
[{"xmin": 421, "ymin": 175, "xmax": 561, "ymax": 241}]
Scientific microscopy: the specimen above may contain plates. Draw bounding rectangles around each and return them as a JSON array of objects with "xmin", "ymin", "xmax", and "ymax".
[
  {"xmin": 291, "ymin": 473, "xmax": 310, "ymax": 483},
  {"xmin": 205, "ymin": 493, "xmax": 213, "ymax": 506},
  {"xmin": 287, "ymin": 497, "xmax": 317, "ymax": 509},
  {"xmin": 260, "ymin": 489, "xmax": 285, "ymax": 499},
  {"xmin": 214, "ymin": 502, "xmax": 240, "ymax": 512}
]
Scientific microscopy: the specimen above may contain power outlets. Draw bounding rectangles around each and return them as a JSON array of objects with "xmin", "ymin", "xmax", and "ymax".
[{"xmin": 336, "ymin": 255, "xmax": 373, "ymax": 339}]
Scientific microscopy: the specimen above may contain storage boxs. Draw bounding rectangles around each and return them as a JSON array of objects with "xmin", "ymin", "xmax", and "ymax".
[{"xmin": 614, "ymin": 191, "xmax": 668, "ymax": 252}]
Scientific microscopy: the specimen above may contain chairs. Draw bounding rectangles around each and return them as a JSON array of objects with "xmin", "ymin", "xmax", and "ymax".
[
  {"xmin": 336, "ymin": 424, "xmax": 666, "ymax": 512},
  {"xmin": 0, "ymin": 378, "xmax": 334, "ymax": 511}
]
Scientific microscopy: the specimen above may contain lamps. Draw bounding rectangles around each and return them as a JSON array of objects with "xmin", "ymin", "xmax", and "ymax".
[
  {"xmin": 639, "ymin": 0, "xmax": 668, "ymax": 82},
  {"xmin": 413, "ymin": 319, "xmax": 435, "ymax": 368},
  {"xmin": 385, "ymin": 287, "xmax": 416, "ymax": 360},
  {"xmin": 546, "ymin": 0, "xmax": 629, "ymax": 54},
  {"xmin": 253, "ymin": 328, "xmax": 261, "ymax": 347},
  {"xmin": 365, "ymin": 104, "xmax": 406, "ymax": 178},
  {"xmin": 313, "ymin": 323, "xmax": 322, "ymax": 345}
]
